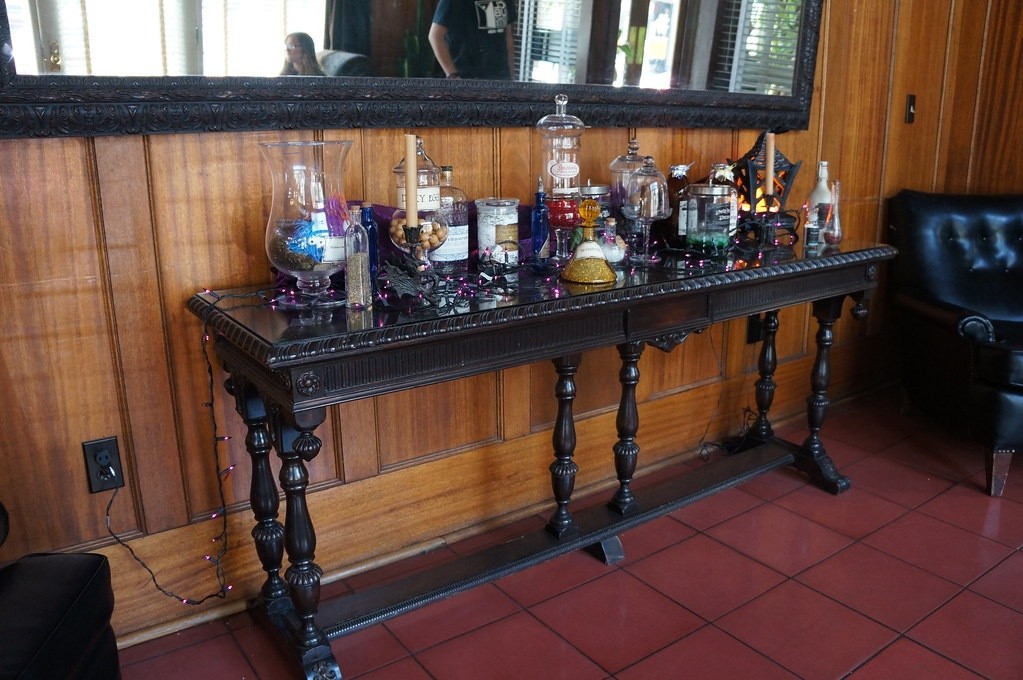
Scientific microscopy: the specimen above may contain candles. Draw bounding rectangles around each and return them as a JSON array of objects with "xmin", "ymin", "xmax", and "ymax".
[
  {"xmin": 765, "ymin": 131, "xmax": 773, "ymax": 195},
  {"xmin": 404, "ymin": 130, "xmax": 419, "ymax": 228}
]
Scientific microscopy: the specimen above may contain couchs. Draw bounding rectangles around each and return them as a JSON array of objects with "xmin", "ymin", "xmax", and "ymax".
[{"xmin": 885, "ymin": 188, "xmax": 1023, "ymax": 498}]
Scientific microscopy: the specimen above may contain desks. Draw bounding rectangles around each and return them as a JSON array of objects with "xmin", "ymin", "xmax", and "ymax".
[{"xmin": 183, "ymin": 237, "xmax": 901, "ymax": 680}]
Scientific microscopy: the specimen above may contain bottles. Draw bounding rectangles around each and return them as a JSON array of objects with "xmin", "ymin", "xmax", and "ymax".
[
  {"xmin": 805, "ymin": 211, "xmax": 819, "ymax": 248},
  {"xmin": 709, "ymin": 164, "xmax": 736, "ymax": 186},
  {"xmin": 344, "ymin": 205, "xmax": 372, "ymax": 309},
  {"xmin": 685, "ymin": 183, "xmax": 737, "ymax": 257},
  {"xmin": 667, "ymin": 164, "xmax": 690, "ymax": 248},
  {"xmin": 548, "ymin": 228, "xmax": 571, "ymax": 281},
  {"xmin": 361, "ymin": 202, "xmax": 378, "ymax": 296},
  {"xmin": 823, "ymin": 180, "xmax": 843, "ymax": 246},
  {"xmin": 811, "ymin": 161, "xmax": 833, "ymax": 244},
  {"xmin": 426, "ymin": 165, "xmax": 470, "ymax": 280},
  {"xmin": 581, "ymin": 179, "xmax": 612, "ymax": 235},
  {"xmin": 531, "ymin": 176, "xmax": 550, "ymax": 274},
  {"xmin": 597, "ymin": 218, "xmax": 625, "ymax": 266}
]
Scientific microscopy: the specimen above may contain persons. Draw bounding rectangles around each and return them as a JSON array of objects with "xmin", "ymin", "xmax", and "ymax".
[
  {"xmin": 428, "ymin": 0, "xmax": 515, "ymax": 80},
  {"xmin": 279, "ymin": 32, "xmax": 325, "ymax": 75}
]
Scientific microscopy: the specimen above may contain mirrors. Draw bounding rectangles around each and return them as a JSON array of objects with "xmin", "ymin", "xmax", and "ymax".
[{"xmin": 0, "ymin": 0, "xmax": 824, "ymax": 140}]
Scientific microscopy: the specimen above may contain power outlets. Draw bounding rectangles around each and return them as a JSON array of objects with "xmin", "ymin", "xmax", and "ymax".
[
  {"xmin": 81, "ymin": 435, "xmax": 125, "ymax": 496},
  {"xmin": 746, "ymin": 312, "xmax": 761, "ymax": 344}
]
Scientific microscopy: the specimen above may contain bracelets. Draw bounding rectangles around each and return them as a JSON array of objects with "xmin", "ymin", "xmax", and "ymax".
[{"xmin": 447, "ymin": 72, "xmax": 460, "ymax": 79}]
